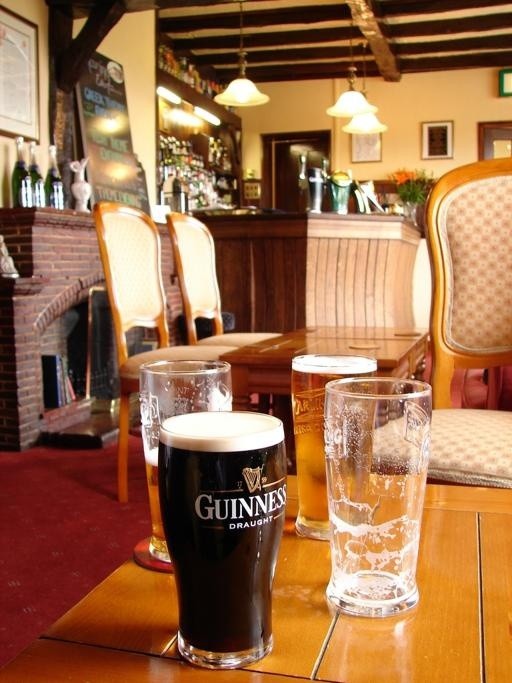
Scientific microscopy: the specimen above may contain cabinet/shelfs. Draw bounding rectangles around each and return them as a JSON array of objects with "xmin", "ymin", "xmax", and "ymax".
[{"xmin": 155, "ymin": 68, "xmax": 244, "ymax": 216}]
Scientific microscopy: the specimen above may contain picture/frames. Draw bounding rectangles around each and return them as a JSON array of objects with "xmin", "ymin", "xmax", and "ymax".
[
  {"xmin": 498, "ymin": 70, "xmax": 511, "ymax": 96},
  {"xmin": 348, "ymin": 134, "xmax": 382, "ymax": 163},
  {"xmin": 420, "ymin": 120, "xmax": 455, "ymax": 161},
  {"xmin": 0, "ymin": 3, "xmax": 42, "ymax": 143}
]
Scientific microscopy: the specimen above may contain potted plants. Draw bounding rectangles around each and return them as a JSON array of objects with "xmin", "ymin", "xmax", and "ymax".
[{"xmin": 391, "ymin": 168, "xmax": 433, "ymax": 224}]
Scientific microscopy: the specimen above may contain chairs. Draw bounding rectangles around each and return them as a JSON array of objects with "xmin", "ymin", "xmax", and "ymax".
[
  {"xmin": 166, "ymin": 211, "xmax": 282, "ymax": 348},
  {"xmin": 372, "ymin": 157, "xmax": 512, "ymax": 491},
  {"xmin": 93, "ymin": 200, "xmax": 241, "ymax": 503}
]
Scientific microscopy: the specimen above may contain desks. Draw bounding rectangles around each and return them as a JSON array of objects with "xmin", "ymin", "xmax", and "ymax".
[
  {"xmin": 1, "ymin": 474, "xmax": 511, "ymax": 681},
  {"xmin": 219, "ymin": 326, "xmax": 431, "ymax": 424}
]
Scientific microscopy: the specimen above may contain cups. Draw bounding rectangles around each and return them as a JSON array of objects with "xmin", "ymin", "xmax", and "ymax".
[
  {"xmin": 141, "ymin": 356, "xmax": 233, "ymax": 565},
  {"xmin": 289, "ymin": 355, "xmax": 379, "ymax": 542},
  {"xmin": 157, "ymin": 410, "xmax": 287, "ymax": 672},
  {"xmin": 308, "ymin": 167, "xmax": 323, "ymax": 214},
  {"xmin": 323, "ymin": 376, "xmax": 433, "ymax": 619}
]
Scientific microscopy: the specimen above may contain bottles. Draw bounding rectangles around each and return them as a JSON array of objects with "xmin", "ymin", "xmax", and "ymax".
[
  {"xmin": 240, "ymin": 169, "xmax": 265, "ymax": 211},
  {"xmin": 321, "ymin": 159, "xmax": 332, "ymax": 212},
  {"xmin": 296, "ymin": 150, "xmax": 311, "ymax": 214},
  {"xmin": 157, "ymin": 42, "xmax": 240, "ymax": 212},
  {"xmin": 12, "ymin": 137, "xmax": 65, "ymax": 210}
]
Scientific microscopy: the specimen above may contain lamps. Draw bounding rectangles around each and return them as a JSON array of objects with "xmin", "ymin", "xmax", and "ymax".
[
  {"xmin": 157, "ymin": 86, "xmax": 181, "ymax": 105},
  {"xmin": 325, "ymin": 2, "xmax": 388, "ymax": 136},
  {"xmin": 194, "ymin": 106, "xmax": 222, "ymax": 125},
  {"xmin": 213, "ymin": 1, "xmax": 270, "ymax": 107}
]
